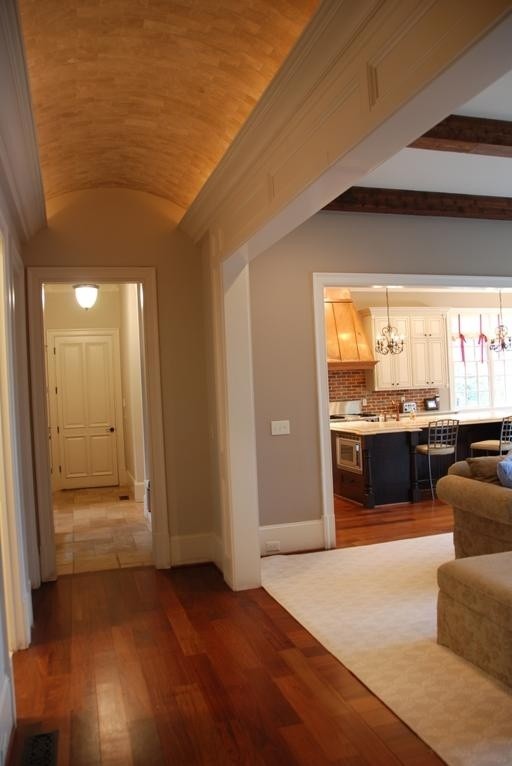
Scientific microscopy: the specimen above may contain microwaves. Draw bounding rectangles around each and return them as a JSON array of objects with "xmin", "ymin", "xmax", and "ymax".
[{"xmin": 338, "ymin": 439, "xmax": 360, "ymax": 469}]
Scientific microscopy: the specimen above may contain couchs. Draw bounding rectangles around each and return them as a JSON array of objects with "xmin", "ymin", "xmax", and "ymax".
[
  {"xmin": 436, "ymin": 453, "xmax": 511, "ymax": 559},
  {"xmin": 437, "ymin": 552, "xmax": 511, "ymax": 689}
]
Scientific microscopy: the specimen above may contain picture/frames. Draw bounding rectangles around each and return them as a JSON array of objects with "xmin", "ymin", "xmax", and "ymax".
[{"xmin": 424, "ymin": 398, "xmax": 438, "ymax": 410}]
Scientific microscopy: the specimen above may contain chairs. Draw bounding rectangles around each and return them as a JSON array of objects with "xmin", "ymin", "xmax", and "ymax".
[
  {"xmin": 412, "ymin": 418, "xmax": 459, "ymax": 503},
  {"xmin": 470, "ymin": 414, "xmax": 512, "ymax": 457}
]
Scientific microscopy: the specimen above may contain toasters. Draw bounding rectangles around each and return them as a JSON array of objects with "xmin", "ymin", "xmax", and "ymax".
[{"xmin": 399, "ymin": 402, "xmax": 416, "ymax": 413}]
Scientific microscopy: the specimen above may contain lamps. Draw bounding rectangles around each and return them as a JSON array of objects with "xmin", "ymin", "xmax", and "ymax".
[
  {"xmin": 489, "ymin": 291, "xmax": 511, "ymax": 352},
  {"xmin": 72, "ymin": 284, "xmax": 99, "ymax": 310},
  {"xmin": 376, "ymin": 287, "xmax": 408, "ymax": 355}
]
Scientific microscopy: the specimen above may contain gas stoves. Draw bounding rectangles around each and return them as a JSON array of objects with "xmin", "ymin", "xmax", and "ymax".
[{"xmin": 330, "ymin": 412, "xmax": 379, "ymax": 423}]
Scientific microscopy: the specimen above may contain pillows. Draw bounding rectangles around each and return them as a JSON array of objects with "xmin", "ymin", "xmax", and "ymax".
[
  {"xmin": 466, "ymin": 455, "xmax": 506, "ymax": 485},
  {"xmin": 496, "ymin": 454, "xmax": 511, "ymax": 488}
]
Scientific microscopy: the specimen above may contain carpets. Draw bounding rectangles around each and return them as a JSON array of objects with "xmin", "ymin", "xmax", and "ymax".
[{"xmin": 257, "ymin": 532, "xmax": 510, "ymax": 766}]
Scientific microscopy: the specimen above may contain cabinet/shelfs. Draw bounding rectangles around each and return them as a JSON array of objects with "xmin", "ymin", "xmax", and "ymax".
[
  {"xmin": 411, "ymin": 307, "xmax": 451, "ymax": 389},
  {"xmin": 356, "ymin": 307, "xmax": 411, "ymax": 391}
]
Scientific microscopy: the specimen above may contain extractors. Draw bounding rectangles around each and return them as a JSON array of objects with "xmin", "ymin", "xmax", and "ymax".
[{"xmin": 324, "ymin": 286, "xmax": 378, "ymax": 371}]
